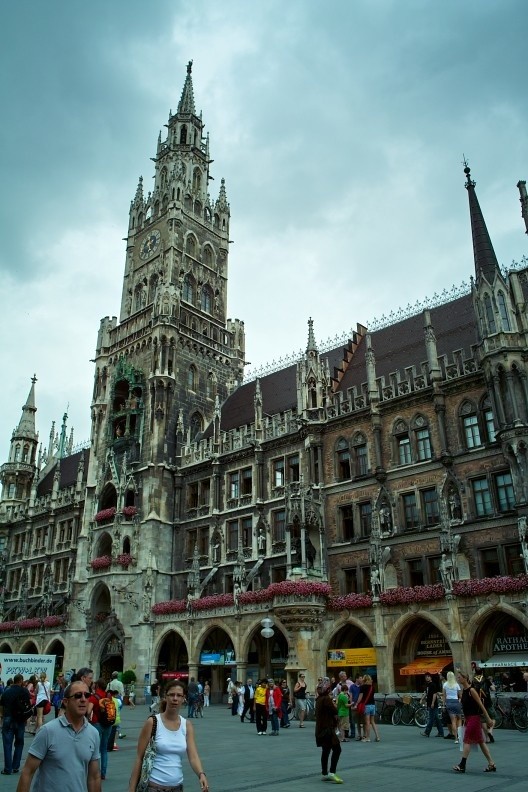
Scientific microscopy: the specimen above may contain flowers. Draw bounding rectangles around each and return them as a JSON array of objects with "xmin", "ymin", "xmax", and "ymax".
[{"xmin": 0, "ymin": 506, "xmax": 528, "ymax": 632}]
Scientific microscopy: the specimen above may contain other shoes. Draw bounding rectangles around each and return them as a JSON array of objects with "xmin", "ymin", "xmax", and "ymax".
[
  {"xmin": 328, "ymin": 773, "xmax": 344, "ymax": 783},
  {"xmin": 272, "ymin": 731, "xmax": 279, "ymax": 736},
  {"xmin": 280, "ymin": 724, "xmax": 290, "ymax": 727},
  {"xmin": 257, "ymin": 732, "xmax": 262, "ymax": 735},
  {"xmin": 101, "ymin": 776, "xmax": 106, "ymax": 781},
  {"xmin": 119, "ymin": 734, "xmax": 126, "ymax": 738},
  {"xmin": 13, "ymin": 768, "xmax": 19, "ymax": 773},
  {"xmin": 300, "ymin": 724, "xmax": 306, "ymax": 728},
  {"xmin": 336, "ymin": 731, "xmax": 380, "ymax": 742},
  {"xmin": 25, "ymin": 729, "xmax": 30, "ymax": 733},
  {"xmin": 113, "ymin": 745, "xmax": 118, "ymax": 750},
  {"xmin": 30, "ymin": 731, "xmax": 36, "ymax": 734},
  {"xmin": 262, "ymin": 731, "xmax": 267, "ymax": 735},
  {"xmin": 1, "ymin": 770, "xmax": 11, "ymax": 775},
  {"xmin": 321, "ymin": 774, "xmax": 332, "ymax": 781},
  {"xmin": 269, "ymin": 732, "xmax": 276, "ymax": 736},
  {"xmin": 419, "ymin": 730, "xmax": 495, "ymax": 744}
]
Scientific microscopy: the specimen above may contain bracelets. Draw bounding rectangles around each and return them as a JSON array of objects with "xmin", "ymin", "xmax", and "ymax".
[{"xmin": 198, "ymin": 773, "xmax": 206, "ymax": 778}]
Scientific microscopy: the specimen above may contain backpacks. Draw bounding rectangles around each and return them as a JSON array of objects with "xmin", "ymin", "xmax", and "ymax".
[{"xmin": 94, "ymin": 694, "xmax": 116, "ymax": 727}]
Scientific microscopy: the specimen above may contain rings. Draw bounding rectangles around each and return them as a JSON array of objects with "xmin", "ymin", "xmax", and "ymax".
[{"xmin": 206, "ymin": 786, "xmax": 210, "ymax": 789}]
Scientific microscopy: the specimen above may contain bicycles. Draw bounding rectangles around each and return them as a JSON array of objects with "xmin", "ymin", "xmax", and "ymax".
[
  {"xmin": 488, "ymin": 694, "xmax": 528, "ymax": 732},
  {"xmin": 306, "ymin": 692, "xmax": 317, "ymax": 720},
  {"xmin": 376, "ymin": 692, "xmax": 448, "ymax": 728},
  {"xmin": 195, "ymin": 693, "xmax": 205, "ymax": 719}
]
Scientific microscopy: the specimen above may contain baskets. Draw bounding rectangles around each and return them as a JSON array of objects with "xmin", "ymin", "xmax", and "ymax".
[{"xmin": 403, "ymin": 695, "xmax": 411, "ymax": 704}]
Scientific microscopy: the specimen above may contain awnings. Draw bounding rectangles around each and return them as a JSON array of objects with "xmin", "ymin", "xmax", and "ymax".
[
  {"xmin": 400, "ymin": 657, "xmax": 453, "ymax": 675},
  {"xmin": 479, "ymin": 653, "xmax": 528, "ymax": 668}
]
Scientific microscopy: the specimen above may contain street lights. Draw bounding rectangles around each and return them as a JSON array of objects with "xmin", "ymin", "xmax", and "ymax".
[{"xmin": 261, "ymin": 617, "xmax": 274, "ymax": 678}]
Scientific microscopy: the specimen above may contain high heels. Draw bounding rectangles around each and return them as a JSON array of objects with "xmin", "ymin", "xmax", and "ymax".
[
  {"xmin": 483, "ymin": 763, "xmax": 497, "ymax": 772},
  {"xmin": 453, "ymin": 766, "xmax": 466, "ymax": 773}
]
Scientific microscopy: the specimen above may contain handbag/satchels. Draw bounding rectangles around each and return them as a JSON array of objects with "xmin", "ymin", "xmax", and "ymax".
[
  {"xmin": 457, "ymin": 724, "xmax": 479, "ymax": 752},
  {"xmin": 129, "ymin": 715, "xmax": 157, "ymax": 792},
  {"xmin": 228, "ymin": 696, "xmax": 233, "ymax": 703},
  {"xmin": 357, "ymin": 703, "xmax": 365, "ymax": 713},
  {"xmin": 43, "ymin": 702, "xmax": 51, "ymax": 715}
]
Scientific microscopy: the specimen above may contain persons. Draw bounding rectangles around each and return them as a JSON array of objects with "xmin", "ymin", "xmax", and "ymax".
[
  {"xmin": 253, "ymin": 678, "xmax": 269, "ymax": 735},
  {"xmin": 420, "ymin": 661, "xmax": 528, "ymax": 773},
  {"xmin": 128, "ymin": 681, "xmax": 209, "ymax": 792},
  {"xmin": 226, "ymin": 673, "xmax": 292, "ymax": 736},
  {"xmin": 0, "ymin": 663, "xmax": 125, "ymax": 792},
  {"xmin": 128, "ymin": 680, "xmax": 136, "ymax": 710},
  {"xmin": 337, "ymin": 685, "xmax": 355, "ymax": 742},
  {"xmin": 314, "ymin": 688, "xmax": 344, "ymax": 783},
  {"xmin": 294, "ymin": 673, "xmax": 308, "ymax": 728},
  {"xmin": 315, "ymin": 670, "xmax": 380, "ymax": 742},
  {"xmin": 149, "ymin": 676, "xmax": 211, "ymax": 718}
]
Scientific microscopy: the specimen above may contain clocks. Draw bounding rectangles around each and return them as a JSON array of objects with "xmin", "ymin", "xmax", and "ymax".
[{"xmin": 139, "ymin": 229, "xmax": 161, "ymax": 261}]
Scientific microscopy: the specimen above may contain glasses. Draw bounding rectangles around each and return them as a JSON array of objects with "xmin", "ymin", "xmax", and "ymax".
[
  {"xmin": 69, "ymin": 692, "xmax": 91, "ymax": 699},
  {"xmin": 281, "ymin": 676, "xmax": 323, "ymax": 683}
]
